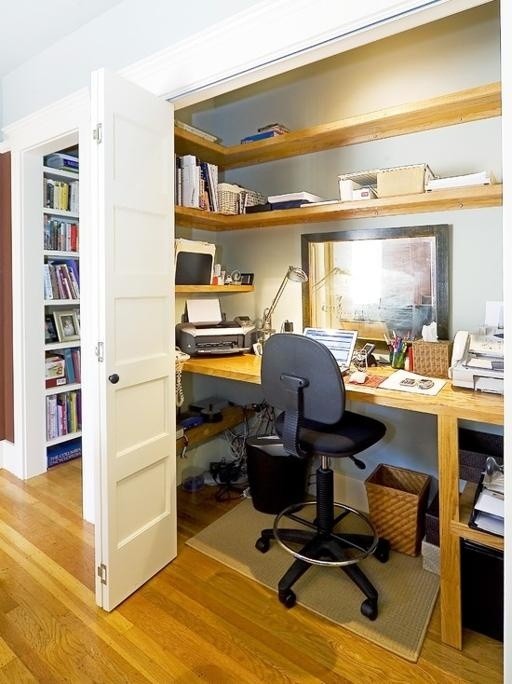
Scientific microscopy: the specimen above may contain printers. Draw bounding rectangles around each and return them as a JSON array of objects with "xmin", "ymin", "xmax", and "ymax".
[
  {"xmin": 451, "ymin": 299, "xmax": 504, "ymax": 395},
  {"xmin": 176, "ymin": 297, "xmax": 256, "ymax": 355}
]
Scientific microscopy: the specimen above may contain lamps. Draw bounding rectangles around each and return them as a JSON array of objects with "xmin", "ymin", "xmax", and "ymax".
[{"xmin": 253, "ymin": 266, "xmax": 308, "ymax": 346}]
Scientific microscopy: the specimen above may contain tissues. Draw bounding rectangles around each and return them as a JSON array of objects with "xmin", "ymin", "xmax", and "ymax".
[{"xmin": 412, "ymin": 321, "xmax": 453, "ymax": 378}]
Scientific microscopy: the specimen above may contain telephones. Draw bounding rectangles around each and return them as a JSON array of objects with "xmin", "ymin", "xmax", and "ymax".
[
  {"xmin": 174, "ymin": 345, "xmax": 190, "ymax": 363},
  {"xmin": 357, "ymin": 342, "xmax": 377, "ymax": 366}
]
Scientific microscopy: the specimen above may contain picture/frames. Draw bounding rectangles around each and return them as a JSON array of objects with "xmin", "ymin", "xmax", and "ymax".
[{"xmin": 300, "ymin": 224, "xmax": 449, "ymax": 348}]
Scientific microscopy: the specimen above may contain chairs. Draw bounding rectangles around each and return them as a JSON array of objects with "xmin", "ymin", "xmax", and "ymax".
[{"xmin": 254, "ymin": 333, "xmax": 392, "ymax": 622}]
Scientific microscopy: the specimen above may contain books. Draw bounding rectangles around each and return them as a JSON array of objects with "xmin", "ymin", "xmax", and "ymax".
[
  {"xmin": 43, "ymin": 178, "xmax": 79, "ymax": 214},
  {"xmin": 44, "ymin": 215, "xmax": 79, "ymax": 252},
  {"xmin": 46, "ymin": 389, "xmax": 82, "ymax": 442},
  {"xmin": 44, "ymin": 260, "xmax": 80, "ymax": 300},
  {"xmin": 426, "ymin": 169, "xmax": 495, "ymax": 190},
  {"xmin": 246, "ymin": 187, "xmax": 340, "ymax": 213},
  {"xmin": 64, "ymin": 349, "xmax": 80, "ymax": 385},
  {"xmin": 177, "ymin": 154, "xmax": 220, "ymax": 213},
  {"xmin": 175, "ymin": 119, "xmax": 224, "ymax": 147},
  {"xmin": 240, "ymin": 123, "xmax": 291, "ymax": 143}
]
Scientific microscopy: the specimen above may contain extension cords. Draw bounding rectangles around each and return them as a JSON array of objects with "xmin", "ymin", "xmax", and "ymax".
[{"xmin": 203, "ymin": 469, "xmax": 247, "ymax": 489}]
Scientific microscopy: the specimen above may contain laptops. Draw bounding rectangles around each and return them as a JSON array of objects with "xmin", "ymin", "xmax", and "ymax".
[{"xmin": 302, "ymin": 327, "xmax": 359, "ymax": 371}]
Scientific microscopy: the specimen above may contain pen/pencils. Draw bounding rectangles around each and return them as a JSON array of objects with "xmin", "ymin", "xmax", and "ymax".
[{"xmin": 384, "ymin": 331, "xmax": 407, "ymax": 370}]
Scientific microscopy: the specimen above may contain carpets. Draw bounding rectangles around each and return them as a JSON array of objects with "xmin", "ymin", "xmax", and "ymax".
[{"xmin": 184, "ymin": 496, "xmax": 440, "ymax": 663}]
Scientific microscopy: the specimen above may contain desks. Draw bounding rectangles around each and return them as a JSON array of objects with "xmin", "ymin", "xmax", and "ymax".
[{"xmin": 175, "ymin": 348, "xmax": 505, "ymax": 651}]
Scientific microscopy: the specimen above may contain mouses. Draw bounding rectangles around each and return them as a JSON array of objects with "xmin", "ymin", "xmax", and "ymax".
[{"xmin": 349, "ymin": 371, "xmax": 368, "ymax": 384}]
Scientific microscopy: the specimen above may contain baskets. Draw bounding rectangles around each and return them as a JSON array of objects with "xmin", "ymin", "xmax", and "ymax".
[{"xmin": 218, "ymin": 190, "xmax": 267, "ymax": 215}]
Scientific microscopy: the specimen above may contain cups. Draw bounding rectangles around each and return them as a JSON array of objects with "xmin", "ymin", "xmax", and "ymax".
[{"xmin": 354, "ymin": 353, "xmax": 367, "ymax": 372}]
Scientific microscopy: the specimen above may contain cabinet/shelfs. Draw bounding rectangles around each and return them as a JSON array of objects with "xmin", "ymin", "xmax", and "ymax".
[
  {"xmin": 174, "ymin": 82, "xmax": 502, "ymax": 293},
  {"xmin": 42, "ymin": 153, "xmax": 83, "ymax": 467}
]
